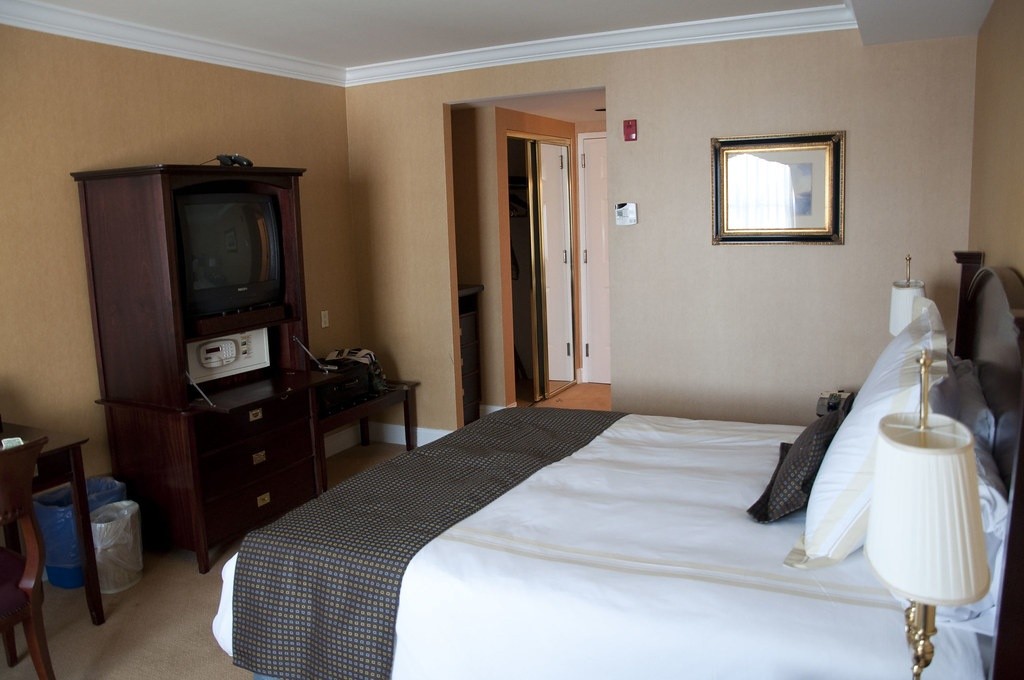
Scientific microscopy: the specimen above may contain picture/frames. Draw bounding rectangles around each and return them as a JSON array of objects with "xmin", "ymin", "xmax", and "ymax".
[{"xmin": 710, "ymin": 130, "xmax": 846, "ymax": 246}]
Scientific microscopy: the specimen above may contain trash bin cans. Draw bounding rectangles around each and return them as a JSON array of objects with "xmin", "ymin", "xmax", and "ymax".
[
  {"xmin": 32, "ymin": 479, "xmax": 129, "ymax": 590},
  {"xmin": 89, "ymin": 499, "xmax": 144, "ymax": 593}
]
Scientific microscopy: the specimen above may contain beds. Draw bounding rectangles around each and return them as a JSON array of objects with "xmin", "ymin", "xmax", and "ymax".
[{"xmin": 212, "ymin": 250, "xmax": 1024, "ymax": 680}]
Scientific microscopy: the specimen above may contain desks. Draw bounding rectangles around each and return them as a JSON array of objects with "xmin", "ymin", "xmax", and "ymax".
[
  {"xmin": 316, "ymin": 378, "xmax": 420, "ymax": 493},
  {"xmin": 0, "ymin": 422, "xmax": 106, "ymax": 627}
]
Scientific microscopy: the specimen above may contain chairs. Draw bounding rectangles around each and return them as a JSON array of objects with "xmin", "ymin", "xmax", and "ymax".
[{"xmin": 0, "ymin": 435, "xmax": 56, "ymax": 680}]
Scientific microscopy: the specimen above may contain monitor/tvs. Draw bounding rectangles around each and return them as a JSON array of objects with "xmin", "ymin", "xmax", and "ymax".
[{"xmin": 179, "ymin": 193, "xmax": 284, "ymax": 318}]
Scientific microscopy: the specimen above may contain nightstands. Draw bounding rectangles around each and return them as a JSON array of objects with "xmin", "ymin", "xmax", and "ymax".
[{"xmin": 815, "ymin": 397, "xmax": 846, "ymax": 417}]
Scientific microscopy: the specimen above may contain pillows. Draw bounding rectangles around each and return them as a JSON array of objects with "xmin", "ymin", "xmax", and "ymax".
[
  {"xmin": 954, "ymin": 358, "xmax": 995, "ymax": 448},
  {"xmin": 889, "ymin": 437, "xmax": 1009, "ymax": 636},
  {"xmin": 744, "ymin": 391, "xmax": 854, "ymax": 524},
  {"xmin": 781, "ymin": 294, "xmax": 950, "ymax": 572}
]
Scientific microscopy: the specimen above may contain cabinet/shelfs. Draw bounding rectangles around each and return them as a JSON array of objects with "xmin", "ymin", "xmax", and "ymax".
[
  {"xmin": 70, "ymin": 165, "xmax": 341, "ymax": 575},
  {"xmin": 458, "ymin": 282, "xmax": 485, "ymax": 427}
]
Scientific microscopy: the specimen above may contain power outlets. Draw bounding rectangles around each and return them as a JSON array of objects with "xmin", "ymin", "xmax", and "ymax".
[{"xmin": 320, "ymin": 310, "xmax": 329, "ymax": 329}]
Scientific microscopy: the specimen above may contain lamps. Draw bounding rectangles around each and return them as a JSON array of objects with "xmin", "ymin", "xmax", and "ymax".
[
  {"xmin": 888, "ymin": 253, "xmax": 926, "ymax": 337},
  {"xmin": 862, "ymin": 347, "xmax": 990, "ymax": 680}
]
